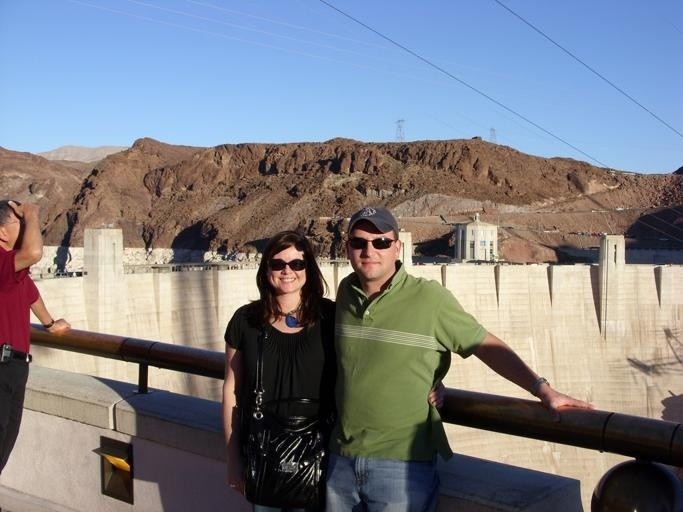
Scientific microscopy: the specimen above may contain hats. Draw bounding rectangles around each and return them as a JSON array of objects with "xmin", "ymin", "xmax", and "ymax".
[{"xmin": 349, "ymin": 206, "xmax": 399, "ymax": 234}]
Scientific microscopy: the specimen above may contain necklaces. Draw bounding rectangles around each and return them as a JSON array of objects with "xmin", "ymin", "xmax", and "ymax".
[{"xmin": 279, "ymin": 307, "xmax": 299, "ymax": 328}]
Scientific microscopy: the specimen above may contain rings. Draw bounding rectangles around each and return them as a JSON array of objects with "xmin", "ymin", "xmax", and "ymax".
[{"xmin": 229, "ymin": 484, "xmax": 236, "ymax": 488}]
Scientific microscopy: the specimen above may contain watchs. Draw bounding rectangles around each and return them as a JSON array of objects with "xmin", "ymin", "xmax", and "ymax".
[{"xmin": 532, "ymin": 378, "xmax": 550, "ymax": 397}]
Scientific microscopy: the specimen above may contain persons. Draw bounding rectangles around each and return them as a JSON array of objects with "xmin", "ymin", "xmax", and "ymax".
[
  {"xmin": 222, "ymin": 231, "xmax": 446, "ymax": 512},
  {"xmin": 0, "ymin": 200, "xmax": 72, "ymax": 473},
  {"xmin": 328, "ymin": 208, "xmax": 595, "ymax": 512}
]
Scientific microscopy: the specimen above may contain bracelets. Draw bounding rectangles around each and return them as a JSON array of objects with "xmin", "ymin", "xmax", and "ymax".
[{"xmin": 44, "ymin": 319, "xmax": 55, "ymax": 329}]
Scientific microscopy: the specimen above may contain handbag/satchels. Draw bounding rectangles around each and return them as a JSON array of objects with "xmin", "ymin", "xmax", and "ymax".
[{"xmin": 245, "ymin": 387, "xmax": 327, "ymax": 508}]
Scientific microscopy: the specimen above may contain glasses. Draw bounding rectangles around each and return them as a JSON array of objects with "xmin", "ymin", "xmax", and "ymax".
[
  {"xmin": 349, "ymin": 237, "xmax": 397, "ymax": 250},
  {"xmin": 267, "ymin": 259, "xmax": 308, "ymax": 271}
]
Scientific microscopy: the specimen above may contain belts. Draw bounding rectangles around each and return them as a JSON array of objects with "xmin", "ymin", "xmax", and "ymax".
[{"xmin": 0, "ymin": 349, "xmax": 33, "ymax": 364}]
